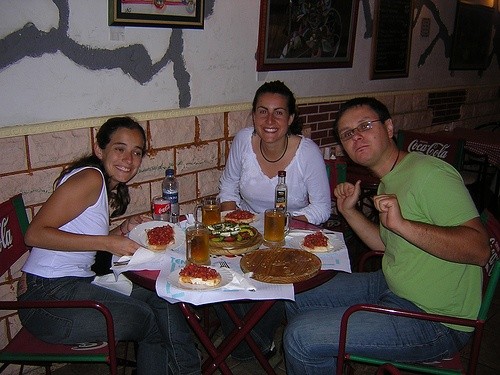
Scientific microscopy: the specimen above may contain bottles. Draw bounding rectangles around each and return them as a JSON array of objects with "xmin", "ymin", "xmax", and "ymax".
[
  {"xmin": 274, "ymin": 171, "xmax": 288, "ymax": 214},
  {"xmin": 161, "ymin": 169, "xmax": 180, "ymax": 226}
]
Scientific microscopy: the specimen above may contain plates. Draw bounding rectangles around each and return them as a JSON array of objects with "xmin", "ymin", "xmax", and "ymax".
[
  {"xmin": 288, "ymin": 235, "xmax": 344, "ymax": 254},
  {"xmin": 129, "ymin": 220, "xmax": 186, "ymax": 252},
  {"xmin": 166, "ymin": 265, "xmax": 233, "ymax": 292},
  {"xmin": 220, "ymin": 209, "xmax": 258, "ymax": 223}
]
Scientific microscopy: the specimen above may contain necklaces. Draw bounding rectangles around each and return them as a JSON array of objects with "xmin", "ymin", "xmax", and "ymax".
[
  {"xmin": 390, "ymin": 148, "xmax": 400, "ymax": 171},
  {"xmin": 260, "ymin": 136, "xmax": 289, "ymax": 163}
]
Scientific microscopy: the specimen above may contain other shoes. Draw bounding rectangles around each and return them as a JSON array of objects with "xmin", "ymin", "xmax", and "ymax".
[{"xmin": 224, "ymin": 355, "xmax": 241, "ymax": 370}]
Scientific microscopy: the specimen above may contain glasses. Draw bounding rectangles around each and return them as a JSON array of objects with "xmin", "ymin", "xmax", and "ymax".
[{"xmin": 341, "ymin": 119, "xmax": 382, "ymax": 143}]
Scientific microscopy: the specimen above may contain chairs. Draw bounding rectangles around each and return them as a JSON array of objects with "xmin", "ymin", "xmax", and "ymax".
[
  {"xmin": 0, "ymin": 193, "xmax": 140, "ymax": 375},
  {"xmin": 325, "ymin": 129, "xmax": 500, "ymax": 375}
]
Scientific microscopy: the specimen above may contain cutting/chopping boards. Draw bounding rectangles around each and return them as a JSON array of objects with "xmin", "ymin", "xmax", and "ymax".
[
  {"xmin": 240, "ymin": 247, "xmax": 323, "ymax": 285},
  {"xmin": 209, "ymin": 231, "xmax": 263, "ymax": 256}
]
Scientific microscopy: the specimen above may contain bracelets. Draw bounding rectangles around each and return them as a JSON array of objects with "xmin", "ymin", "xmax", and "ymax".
[{"xmin": 120, "ymin": 224, "xmax": 126, "ymax": 234}]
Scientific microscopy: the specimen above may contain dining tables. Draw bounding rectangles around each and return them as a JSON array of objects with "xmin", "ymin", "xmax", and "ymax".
[{"xmin": 110, "ymin": 210, "xmax": 352, "ymax": 375}]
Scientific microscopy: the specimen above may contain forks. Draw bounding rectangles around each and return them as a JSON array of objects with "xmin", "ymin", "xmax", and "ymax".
[{"xmin": 220, "ymin": 261, "xmax": 231, "ymax": 268}]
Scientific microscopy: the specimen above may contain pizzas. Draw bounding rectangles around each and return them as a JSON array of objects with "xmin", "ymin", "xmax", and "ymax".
[
  {"xmin": 224, "ymin": 210, "xmax": 256, "ymax": 223},
  {"xmin": 302, "ymin": 232, "xmax": 334, "ymax": 251},
  {"xmin": 179, "ymin": 262, "xmax": 221, "ymax": 285},
  {"xmin": 146, "ymin": 224, "xmax": 176, "ymax": 250}
]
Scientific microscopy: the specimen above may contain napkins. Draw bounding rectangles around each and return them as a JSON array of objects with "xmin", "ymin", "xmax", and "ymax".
[
  {"xmin": 221, "ymin": 266, "xmax": 256, "ymax": 291},
  {"xmin": 91, "ymin": 273, "xmax": 133, "ymax": 296},
  {"xmin": 109, "ymin": 247, "xmax": 160, "ymax": 282}
]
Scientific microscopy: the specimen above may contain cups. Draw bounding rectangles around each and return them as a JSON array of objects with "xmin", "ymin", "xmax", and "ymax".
[
  {"xmin": 194, "ymin": 200, "xmax": 222, "ymax": 226},
  {"xmin": 185, "ymin": 225, "xmax": 211, "ymax": 267},
  {"xmin": 151, "ymin": 195, "xmax": 171, "ymax": 222},
  {"xmin": 263, "ymin": 208, "xmax": 292, "ymax": 248}
]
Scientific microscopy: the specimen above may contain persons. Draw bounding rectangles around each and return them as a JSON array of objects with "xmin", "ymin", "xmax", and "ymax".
[
  {"xmin": 281, "ymin": 96, "xmax": 488, "ymax": 375},
  {"xmin": 216, "ymin": 80, "xmax": 333, "ymax": 360},
  {"xmin": 17, "ymin": 117, "xmax": 202, "ymax": 375}
]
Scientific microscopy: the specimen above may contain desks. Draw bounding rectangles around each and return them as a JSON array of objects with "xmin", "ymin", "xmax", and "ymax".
[{"xmin": 462, "ymin": 131, "xmax": 500, "ymax": 198}]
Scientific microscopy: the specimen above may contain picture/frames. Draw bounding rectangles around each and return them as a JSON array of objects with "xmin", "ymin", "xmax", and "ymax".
[
  {"xmin": 108, "ymin": 0, "xmax": 205, "ymax": 30},
  {"xmin": 256, "ymin": 0, "xmax": 360, "ymax": 72},
  {"xmin": 369, "ymin": 0, "xmax": 415, "ymax": 80}
]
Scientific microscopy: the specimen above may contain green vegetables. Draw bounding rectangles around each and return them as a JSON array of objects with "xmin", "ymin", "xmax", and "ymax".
[{"xmin": 203, "ymin": 221, "xmax": 241, "ymax": 238}]
